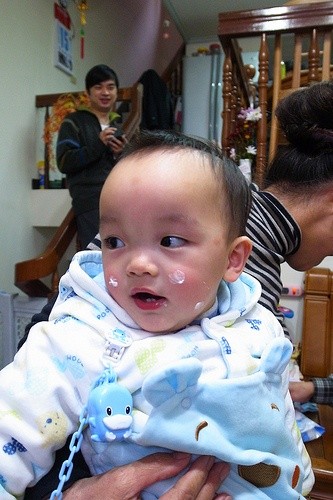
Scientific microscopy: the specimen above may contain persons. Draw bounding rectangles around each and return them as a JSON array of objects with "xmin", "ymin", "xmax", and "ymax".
[
  {"xmin": 288, "ymin": 373, "xmax": 333, "ymax": 408},
  {"xmin": 17, "ymin": 80, "xmax": 333, "ymax": 500},
  {"xmin": 57, "ymin": 63, "xmax": 137, "ymax": 251},
  {"xmin": 0, "ymin": 130, "xmax": 315, "ymax": 500}
]
